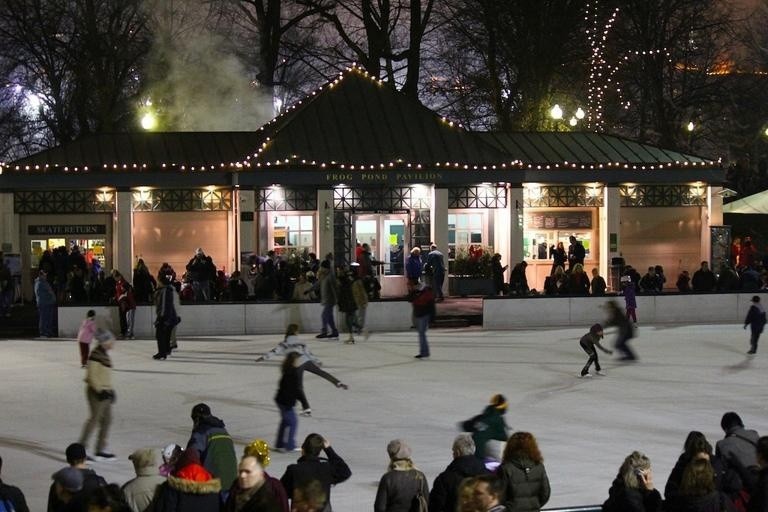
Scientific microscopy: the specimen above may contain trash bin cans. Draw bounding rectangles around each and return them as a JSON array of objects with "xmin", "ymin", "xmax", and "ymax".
[
  {"xmin": 241, "ymin": 251, "xmax": 258, "ymax": 296},
  {"xmin": 609, "ymin": 256, "xmax": 625, "ymax": 294}
]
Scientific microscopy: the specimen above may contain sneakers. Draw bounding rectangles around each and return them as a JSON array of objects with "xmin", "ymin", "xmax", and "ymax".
[
  {"xmin": 40, "ymin": 331, "xmax": 50, "ymax": 337},
  {"xmin": 154, "ymin": 353, "xmax": 166, "ymax": 359}
]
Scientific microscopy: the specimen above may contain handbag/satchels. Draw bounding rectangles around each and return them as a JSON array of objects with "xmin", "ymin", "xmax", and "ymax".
[
  {"xmin": 417, "ymin": 493, "xmax": 427, "ymax": 512},
  {"xmin": 291, "ymin": 480, "xmax": 329, "ymax": 511}
]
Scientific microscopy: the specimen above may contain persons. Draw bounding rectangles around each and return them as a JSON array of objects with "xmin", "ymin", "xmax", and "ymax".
[
  {"xmin": 274, "ymin": 351, "xmax": 303, "ymax": 453},
  {"xmin": 247, "ymin": 243, "xmax": 381, "ymax": 338},
  {"xmin": 151, "ymin": 276, "xmax": 182, "ymax": 361},
  {"xmin": 619, "ymin": 275, "xmax": 638, "ymax": 328},
  {"xmin": 0, "ymin": 453, "xmax": 31, "ymax": 512},
  {"xmin": 34, "ymin": 245, "xmax": 136, "ymax": 340},
  {"xmin": 46, "ymin": 402, "xmax": 352, "ymax": 512},
  {"xmin": 743, "ymin": 295, "xmax": 766, "ymax": 354},
  {"xmin": 601, "ymin": 451, "xmax": 663, "ymax": 512},
  {"xmin": 77, "ymin": 327, "xmax": 118, "ymax": 464},
  {"xmin": 662, "ymin": 411, "xmax": 768, "ymax": 512},
  {"xmin": 374, "ymin": 394, "xmax": 551, "ymax": 511},
  {"xmin": 578, "ymin": 323, "xmax": 613, "ymax": 378},
  {"xmin": 605, "ymin": 302, "xmax": 636, "ymax": 360},
  {"xmin": 491, "ymin": 235, "xmax": 666, "ymax": 298},
  {"xmin": 131, "ymin": 248, "xmax": 249, "ymax": 303},
  {"xmin": 255, "ymin": 324, "xmax": 349, "ymax": 416},
  {"xmin": 676, "ymin": 236, "xmax": 767, "ymax": 293},
  {"xmin": 405, "ymin": 243, "xmax": 445, "ymax": 358}
]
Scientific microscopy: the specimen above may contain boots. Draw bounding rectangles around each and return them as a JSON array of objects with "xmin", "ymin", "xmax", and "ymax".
[
  {"xmin": 316, "ymin": 333, "xmax": 328, "ymax": 338},
  {"xmin": 328, "ymin": 330, "xmax": 338, "ymax": 337}
]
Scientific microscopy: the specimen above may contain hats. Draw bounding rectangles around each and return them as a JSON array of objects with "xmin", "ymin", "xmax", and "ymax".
[
  {"xmin": 121, "ymin": 281, "xmax": 129, "ymax": 291},
  {"xmin": 174, "ymin": 449, "xmax": 201, "ymax": 467},
  {"xmin": 288, "ymin": 352, "xmax": 304, "ymax": 360},
  {"xmin": 95, "ymin": 328, "xmax": 112, "ymax": 343},
  {"xmin": 51, "ymin": 444, "xmax": 86, "ymax": 492},
  {"xmin": 320, "ymin": 259, "xmax": 330, "ymax": 268},
  {"xmin": 388, "ymin": 440, "xmax": 411, "ymax": 459},
  {"xmin": 191, "ymin": 403, "xmax": 212, "ymax": 421}
]
